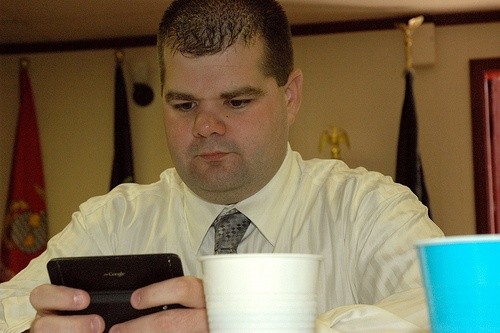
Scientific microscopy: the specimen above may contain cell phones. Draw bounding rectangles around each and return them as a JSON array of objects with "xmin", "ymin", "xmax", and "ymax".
[{"xmin": 47, "ymin": 254, "xmax": 184, "ymax": 333}]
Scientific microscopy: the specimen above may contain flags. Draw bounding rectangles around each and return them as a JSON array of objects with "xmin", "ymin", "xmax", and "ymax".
[
  {"xmin": 395, "ymin": 69, "xmax": 434, "ymax": 221},
  {"xmin": 108, "ymin": 60, "xmax": 135, "ymax": 191}
]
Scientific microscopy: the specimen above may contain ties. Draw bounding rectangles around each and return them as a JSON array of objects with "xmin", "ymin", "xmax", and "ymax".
[{"xmin": 211, "ymin": 211, "xmax": 252, "ymax": 255}]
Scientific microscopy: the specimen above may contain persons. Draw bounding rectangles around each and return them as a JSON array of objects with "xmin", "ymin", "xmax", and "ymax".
[
  {"xmin": 0, "ymin": 0, "xmax": 447, "ymax": 333},
  {"xmin": 0, "ymin": 66, "xmax": 49, "ymax": 285}
]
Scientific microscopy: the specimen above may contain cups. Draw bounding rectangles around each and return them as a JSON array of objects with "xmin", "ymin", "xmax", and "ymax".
[
  {"xmin": 198, "ymin": 253, "xmax": 322, "ymax": 333},
  {"xmin": 415, "ymin": 234, "xmax": 500, "ymax": 333}
]
